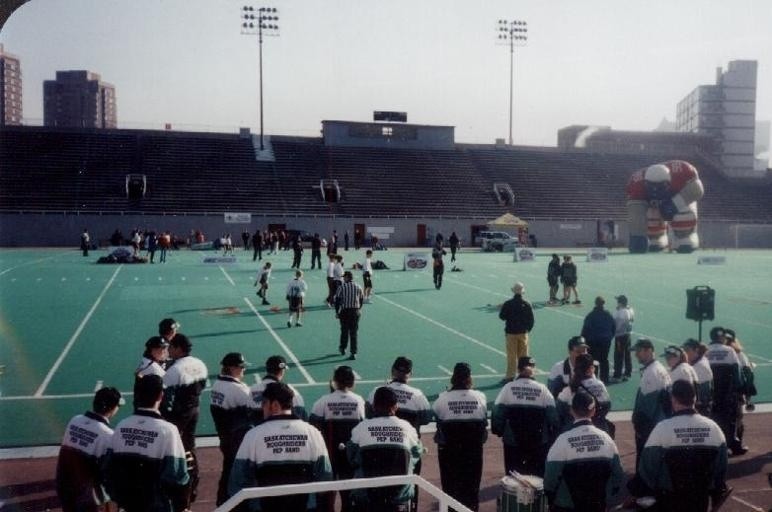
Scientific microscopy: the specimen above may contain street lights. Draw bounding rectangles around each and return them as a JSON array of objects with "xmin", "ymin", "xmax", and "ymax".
[
  {"xmin": 239, "ymin": 4, "xmax": 281, "ymax": 148},
  {"xmin": 494, "ymin": 17, "xmax": 528, "ymax": 146}
]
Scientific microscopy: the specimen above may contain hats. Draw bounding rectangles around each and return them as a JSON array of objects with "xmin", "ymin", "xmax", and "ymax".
[
  {"xmin": 511, "ymin": 283, "xmax": 524, "ymax": 293},
  {"xmin": 340, "ymin": 272, "xmax": 351, "ymax": 277},
  {"xmin": 615, "ymin": 295, "xmax": 627, "ymax": 306}
]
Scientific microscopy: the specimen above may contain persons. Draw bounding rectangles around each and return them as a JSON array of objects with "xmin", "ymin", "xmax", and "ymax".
[
  {"xmin": 434, "ymin": 335, "xmax": 629, "ymax": 512},
  {"xmin": 630, "ymin": 326, "xmax": 758, "ymax": 511},
  {"xmin": 578, "ymin": 295, "xmax": 616, "ymax": 385},
  {"xmin": 282, "ymin": 268, "xmax": 308, "ymax": 327},
  {"xmin": 217, "ymin": 227, "xmax": 290, "ymax": 262},
  {"xmin": 48, "ymin": 383, "xmax": 126, "ymax": 511},
  {"xmin": 332, "ymin": 271, "xmax": 364, "ymax": 360},
  {"xmin": 447, "ymin": 231, "xmax": 459, "ymax": 263},
  {"xmin": 610, "ymin": 295, "xmax": 636, "ymax": 383},
  {"xmin": 210, "ymin": 352, "xmax": 434, "ymax": 512},
  {"xmin": 358, "ymin": 249, "xmax": 374, "ymax": 304},
  {"xmin": 310, "ymin": 232, "xmax": 322, "ymax": 270},
  {"xmin": 252, "ymin": 261, "xmax": 274, "ymax": 305},
  {"xmin": 544, "ymin": 252, "xmax": 581, "ymax": 307},
  {"xmin": 499, "ymin": 281, "xmax": 539, "ymax": 382},
  {"xmin": 432, "ymin": 242, "xmax": 447, "ymax": 290},
  {"xmin": 135, "ymin": 318, "xmax": 208, "ymax": 502},
  {"xmin": 111, "ymin": 226, "xmax": 205, "ymax": 269},
  {"xmin": 105, "ymin": 374, "xmax": 196, "ymax": 512},
  {"xmin": 291, "ymin": 235, "xmax": 304, "ymax": 268},
  {"xmin": 325, "ymin": 252, "xmax": 345, "ymax": 307},
  {"xmin": 327, "ymin": 229, "xmax": 361, "ymax": 257},
  {"xmin": 82, "ymin": 228, "xmax": 90, "ymax": 257}
]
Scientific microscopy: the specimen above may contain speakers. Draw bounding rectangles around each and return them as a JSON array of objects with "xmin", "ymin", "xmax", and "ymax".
[{"xmin": 685, "ymin": 286, "xmax": 715, "ymax": 321}]
